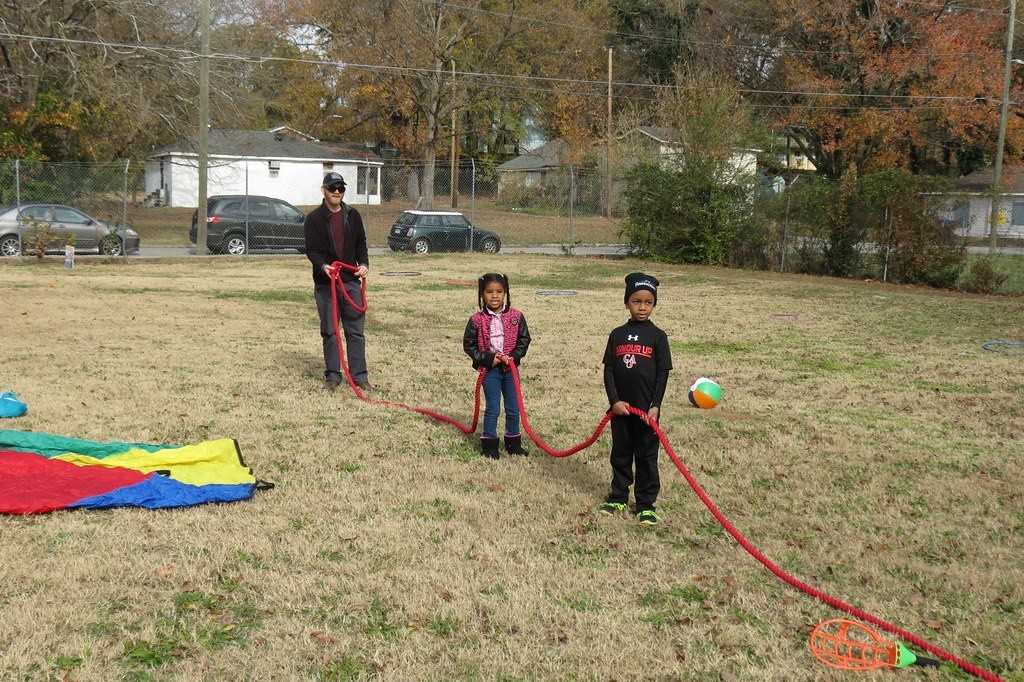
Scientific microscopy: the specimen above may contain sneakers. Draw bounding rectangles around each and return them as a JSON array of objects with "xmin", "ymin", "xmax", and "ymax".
[
  {"xmin": 637, "ymin": 507, "xmax": 661, "ymax": 525},
  {"xmin": 599, "ymin": 502, "xmax": 628, "ymax": 517}
]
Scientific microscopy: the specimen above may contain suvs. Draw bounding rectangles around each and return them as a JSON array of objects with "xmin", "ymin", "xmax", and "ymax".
[
  {"xmin": 189, "ymin": 194, "xmax": 309, "ymax": 256},
  {"xmin": 388, "ymin": 210, "xmax": 502, "ymax": 256}
]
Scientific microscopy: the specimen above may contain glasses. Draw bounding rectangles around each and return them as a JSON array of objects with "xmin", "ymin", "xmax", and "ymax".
[{"xmin": 327, "ymin": 186, "xmax": 346, "ymax": 192}]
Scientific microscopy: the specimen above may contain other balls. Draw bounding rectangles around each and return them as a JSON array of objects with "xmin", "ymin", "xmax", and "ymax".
[{"xmin": 688, "ymin": 377, "xmax": 722, "ymax": 409}]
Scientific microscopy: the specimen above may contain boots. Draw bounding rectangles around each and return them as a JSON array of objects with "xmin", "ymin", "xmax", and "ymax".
[
  {"xmin": 504, "ymin": 432, "xmax": 529, "ymax": 456},
  {"xmin": 480, "ymin": 435, "xmax": 500, "ymax": 460}
]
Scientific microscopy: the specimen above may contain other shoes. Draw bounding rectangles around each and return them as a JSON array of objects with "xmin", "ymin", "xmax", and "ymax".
[
  {"xmin": 356, "ymin": 381, "xmax": 373, "ymax": 392},
  {"xmin": 326, "ymin": 380, "xmax": 338, "ymax": 390}
]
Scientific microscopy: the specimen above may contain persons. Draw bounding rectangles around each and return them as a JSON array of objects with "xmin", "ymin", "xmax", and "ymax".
[
  {"xmin": 303, "ymin": 173, "xmax": 376, "ymax": 393},
  {"xmin": 462, "ymin": 273, "xmax": 532, "ymax": 459},
  {"xmin": 600, "ymin": 272, "xmax": 672, "ymax": 525}
]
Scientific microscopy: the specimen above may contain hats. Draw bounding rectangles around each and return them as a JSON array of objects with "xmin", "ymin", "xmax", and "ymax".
[
  {"xmin": 624, "ymin": 273, "xmax": 659, "ymax": 307},
  {"xmin": 323, "ymin": 172, "xmax": 347, "ymax": 186}
]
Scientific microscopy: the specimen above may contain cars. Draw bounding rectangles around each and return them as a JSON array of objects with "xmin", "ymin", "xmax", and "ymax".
[{"xmin": 0, "ymin": 203, "xmax": 141, "ymax": 257}]
